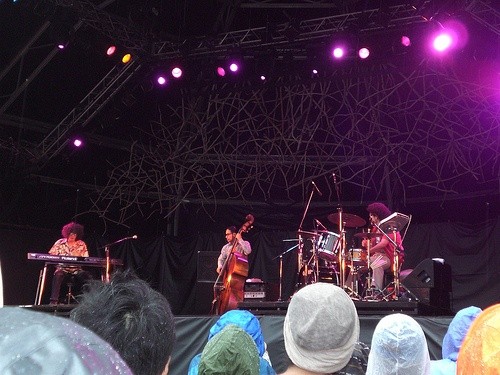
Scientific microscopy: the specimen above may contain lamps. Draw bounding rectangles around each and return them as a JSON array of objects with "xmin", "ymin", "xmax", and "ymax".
[{"xmin": 57, "ymin": 36, "xmax": 70, "ymax": 49}]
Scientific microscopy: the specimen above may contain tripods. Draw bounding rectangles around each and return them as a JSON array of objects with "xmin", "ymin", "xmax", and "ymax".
[{"xmin": 345, "ymin": 219, "xmax": 419, "ymax": 301}]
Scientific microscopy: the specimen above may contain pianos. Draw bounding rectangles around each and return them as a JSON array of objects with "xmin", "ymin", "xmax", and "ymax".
[{"xmin": 28, "ymin": 253, "xmax": 124, "ymax": 308}]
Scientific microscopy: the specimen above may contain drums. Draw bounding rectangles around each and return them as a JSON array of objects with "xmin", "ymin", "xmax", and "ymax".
[
  {"xmin": 309, "ymin": 230, "xmax": 341, "ymax": 261},
  {"xmin": 294, "ymin": 264, "xmax": 339, "ymax": 295},
  {"xmin": 347, "ymin": 249, "xmax": 365, "ymax": 264}
]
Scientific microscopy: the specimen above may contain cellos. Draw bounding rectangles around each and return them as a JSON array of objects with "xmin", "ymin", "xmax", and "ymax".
[{"xmin": 208, "ymin": 215, "xmax": 254, "ymax": 315}]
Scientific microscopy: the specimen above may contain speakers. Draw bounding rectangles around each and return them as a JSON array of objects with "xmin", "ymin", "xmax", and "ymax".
[{"xmin": 401, "ymin": 258, "xmax": 451, "ymax": 292}]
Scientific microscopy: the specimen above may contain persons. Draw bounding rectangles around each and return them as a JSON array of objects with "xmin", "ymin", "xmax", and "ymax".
[
  {"xmin": 365, "ymin": 312, "xmax": 431, "ymax": 375},
  {"xmin": 198, "ymin": 324, "xmax": 260, "ymax": 375},
  {"xmin": 427, "ymin": 306, "xmax": 482, "ymax": 375},
  {"xmin": 457, "ymin": 303, "xmax": 500, "ymax": 375},
  {"xmin": 345, "ymin": 202, "xmax": 404, "ymax": 300},
  {"xmin": 71, "ymin": 269, "xmax": 175, "ymax": 375},
  {"xmin": 188, "ymin": 309, "xmax": 276, "ymax": 375},
  {"xmin": 48, "ymin": 222, "xmax": 93, "ymax": 305},
  {"xmin": 0, "ymin": 305, "xmax": 134, "ymax": 375},
  {"xmin": 277, "ymin": 282, "xmax": 360, "ymax": 375},
  {"xmin": 216, "ymin": 226, "xmax": 251, "ymax": 275}
]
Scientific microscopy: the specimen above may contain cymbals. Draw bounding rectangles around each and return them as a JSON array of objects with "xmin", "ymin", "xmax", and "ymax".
[
  {"xmin": 296, "ymin": 231, "xmax": 321, "ymax": 238},
  {"xmin": 354, "ymin": 232, "xmax": 383, "ymax": 239},
  {"xmin": 327, "ymin": 213, "xmax": 366, "ymax": 227}
]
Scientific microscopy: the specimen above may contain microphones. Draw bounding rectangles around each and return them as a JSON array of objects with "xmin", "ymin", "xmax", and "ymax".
[
  {"xmin": 315, "ymin": 219, "xmax": 327, "ymax": 229},
  {"xmin": 312, "ymin": 180, "xmax": 322, "ymax": 196},
  {"xmin": 127, "ymin": 235, "xmax": 137, "ymax": 239}
]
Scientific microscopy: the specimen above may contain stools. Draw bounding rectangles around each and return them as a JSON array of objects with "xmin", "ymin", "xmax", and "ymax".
[{"xmin": 64, "ymin": 279, "xmax": 78, "ymax": 304}]
